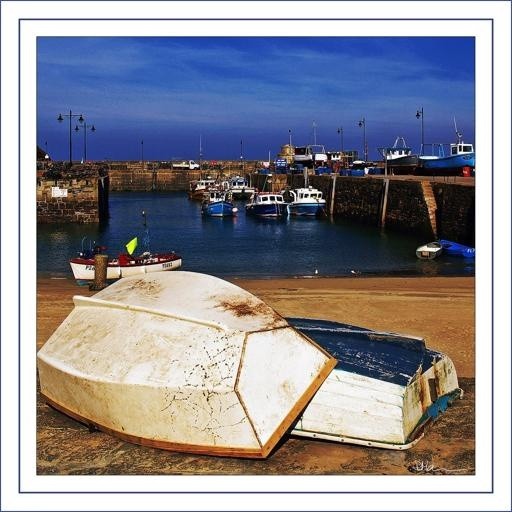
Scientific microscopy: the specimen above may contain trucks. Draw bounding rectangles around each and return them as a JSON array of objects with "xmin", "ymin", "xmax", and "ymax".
[{"xmin": 173, "ymin": 160, "xmax": 200, "ymax": 169}]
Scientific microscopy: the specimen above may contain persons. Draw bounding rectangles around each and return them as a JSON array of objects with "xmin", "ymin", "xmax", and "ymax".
[
  {"xmin": 285, "ymin": 187, "xmax": 289, "ymax": 197},
  {"xmin": 335, "ymin": 160, "xmax": 340, "ymax": 173},
  {"xmin": 199, "ymin": 193, "xmax": 209, "ymax": 199},
  {"xmin": 117, "ymin": 253, "xmax": 132, "ymax": 266},
  {"xmin": 191, "ymin": 184, "xmax": 196, "ymax": 193},
  {"xmin": 247, "ymin": 193, "xmax": 258, "ymax": 204}
]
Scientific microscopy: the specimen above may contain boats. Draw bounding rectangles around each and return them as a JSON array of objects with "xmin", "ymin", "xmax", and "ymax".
[
  {"xmin": 415, "ymin": 239, "xmax": 475, "ymax": 261},
  {"xmin": 69, "ymin": 252, "xmax": 182, "ymax": 281},
  {"xmin": 187, "ymin": 175, "xmax": 328, "ymax": 220},
  {"xmin": 293, "ymin": 132, "xmax": 475, "ymax": 176}
]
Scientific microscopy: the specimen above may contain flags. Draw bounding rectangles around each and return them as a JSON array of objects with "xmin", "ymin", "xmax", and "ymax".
[{"xmin": 126, "ymin": 237, "xmax": 137, "ymax": 255}]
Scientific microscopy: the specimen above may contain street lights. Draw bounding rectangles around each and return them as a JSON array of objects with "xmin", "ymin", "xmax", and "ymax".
[
  {"xmin": 141, "ymin": 139, "xmax": 144, "ymax": 161},
  {"xmin": 240, "ymin": 140, "xmax": 242, "ymax": 162},
  {"xmin": 415, "ymin": 108, "xmax": 424, "ymax": 154},
  {"xmin": 336, "ymin": 117, "xmax": 368, "ymax": 162},
  {"xmin": 57, "ymin": 109, "xmax": 96, "ymax": 166}
]
